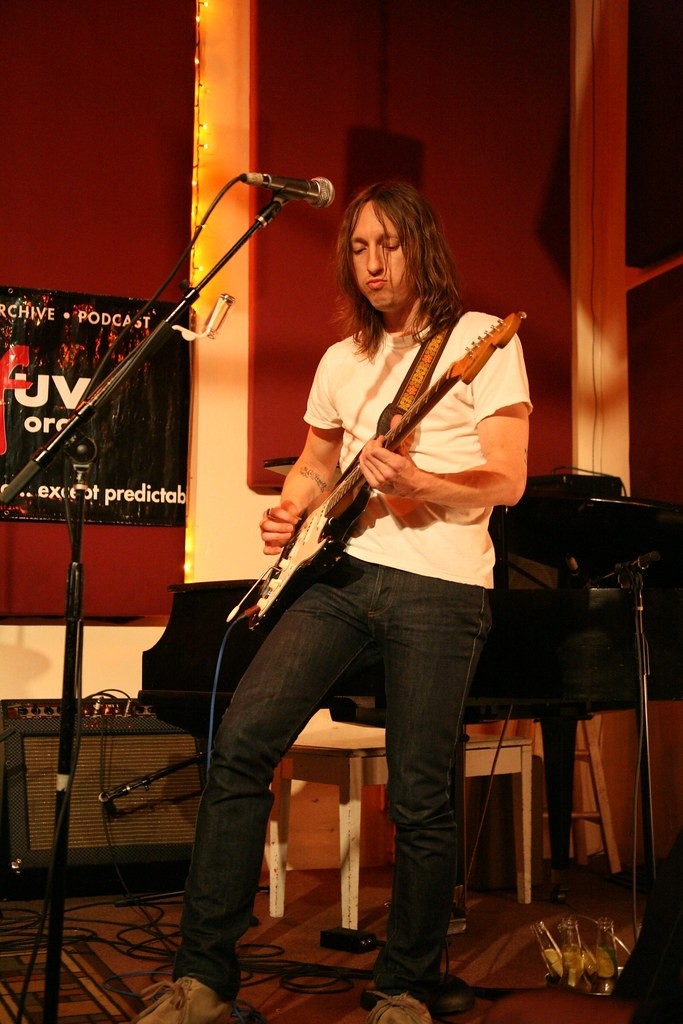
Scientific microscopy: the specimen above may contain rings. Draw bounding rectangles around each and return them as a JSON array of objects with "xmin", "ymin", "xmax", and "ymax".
[{"xmin": 267, "ymin": 508, "xmax": 277, "ymax": 520}]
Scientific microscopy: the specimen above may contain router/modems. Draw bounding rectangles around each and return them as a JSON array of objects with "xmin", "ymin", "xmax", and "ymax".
[{"xmin": 320, "ymin": 926, "xmax": 378, "ymax": 952}]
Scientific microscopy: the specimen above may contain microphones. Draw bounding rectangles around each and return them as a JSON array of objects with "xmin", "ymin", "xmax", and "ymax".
[
  {"xmin": 238, "ymin": 174, "xmax": 336, "ymax": 208},
  {"xmin": 98, "ymin": 790, "xmax": 117, "ymax": 817},
  {"xmin": 563, "ymin": 553, "xmax": 592, "ymax": 589}
]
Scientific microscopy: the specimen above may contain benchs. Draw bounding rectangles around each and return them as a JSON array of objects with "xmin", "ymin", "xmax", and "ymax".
[{"xmin": 268, "ymin": 731, "xmax": 534, "ymax": 931}]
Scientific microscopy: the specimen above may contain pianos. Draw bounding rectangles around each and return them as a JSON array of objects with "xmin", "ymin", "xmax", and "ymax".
[{"xmin": 136, "ymin": 473, "xmax": 683, "ymax": 935}]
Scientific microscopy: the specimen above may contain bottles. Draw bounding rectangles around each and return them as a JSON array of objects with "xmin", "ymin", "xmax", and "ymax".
[
  {"xmin": 557, "ymin": 922, "xmax": 597, "ymax": 993},
  {"xmin": 530, "ymin": 920, "xmax": 563, "ymax": 986},
  {"xmin": 557, "ymin": 915, "xmax": 591, "ymax": 994},
  {"xmin": 591, "ymin": 916, "xmax": 620, "ymax": 994}
]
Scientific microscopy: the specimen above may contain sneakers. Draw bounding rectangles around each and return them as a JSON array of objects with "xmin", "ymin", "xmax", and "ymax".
[
  {"xmin": 364, "ymin": 988, "xmax": 434, "ymax": 1024},
  {"xmin": 130, "ymin": 979, "xmax": 233, "ymax": 1024}
]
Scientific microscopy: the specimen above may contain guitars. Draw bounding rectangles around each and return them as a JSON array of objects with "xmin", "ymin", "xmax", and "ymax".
[{"xmin": 248, "ymin": 310, "xmax": 527, "ymax": 634}]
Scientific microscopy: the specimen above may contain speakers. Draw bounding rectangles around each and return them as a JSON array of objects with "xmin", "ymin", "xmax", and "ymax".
[
  {"xmin": 0, "ymin": 713, "xmax": 217, "ymax": 900},
  {"xmin": 474, "ymin": 493, "xmax": 683, "ymax": 700}
]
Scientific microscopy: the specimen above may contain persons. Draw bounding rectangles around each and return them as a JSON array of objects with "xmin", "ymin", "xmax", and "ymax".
[{"xmin": 133, "ymin": 178, "xmax": 534, "ymax": 1024}]
